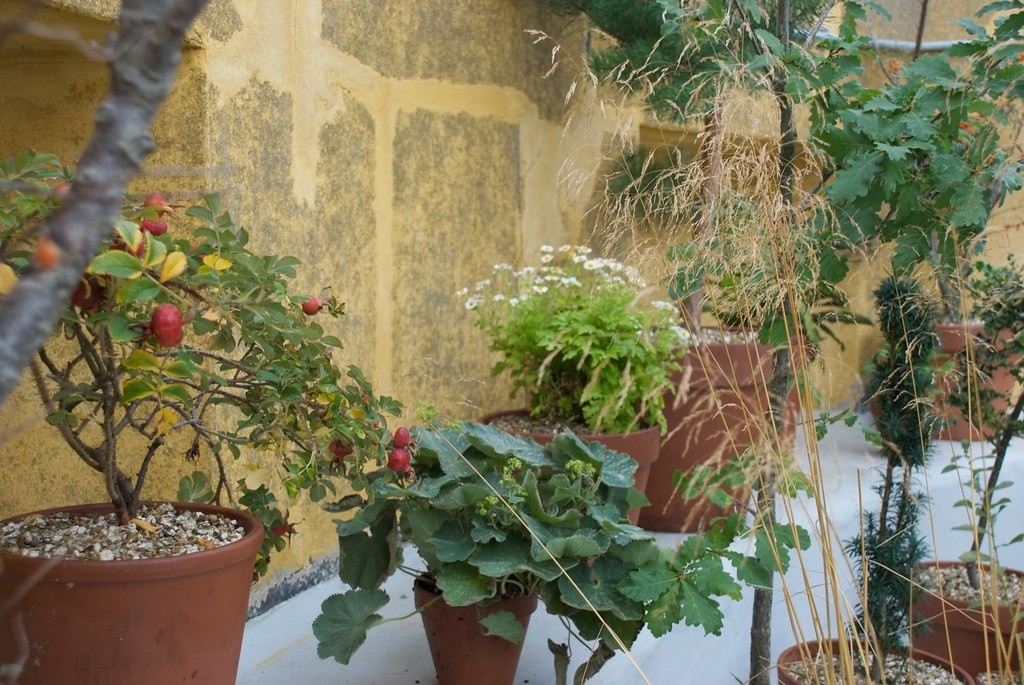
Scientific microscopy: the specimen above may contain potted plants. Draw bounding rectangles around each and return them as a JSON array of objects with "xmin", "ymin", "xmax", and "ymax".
[
  {"xmin": 566, "ymin": 1, "xmax": 831, "ymax": 536},
  {"xmin": 0, "ymin": 145, "xmax": 410, "ymax": 685},
  {"xmin": 778, "ymin": 255, "xmax": 1024, "ymax": 685},
  {"xmin": 798, "ymin": 1, "xmax": 1024, "ymax": 446},
  {"xmin": 312, "ymin": 420, "xmax": 812, "ymax": 685}
]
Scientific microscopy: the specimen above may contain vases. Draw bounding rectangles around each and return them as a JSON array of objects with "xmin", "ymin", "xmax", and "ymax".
[{"xmin": 474, "ymin": 411, "xmax": 659, "ymax": 530}]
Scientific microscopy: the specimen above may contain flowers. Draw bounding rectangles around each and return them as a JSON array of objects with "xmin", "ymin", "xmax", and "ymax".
[{"xmin": 456, "ymin": 242, "xmax": 694, "ymax": 436}]
